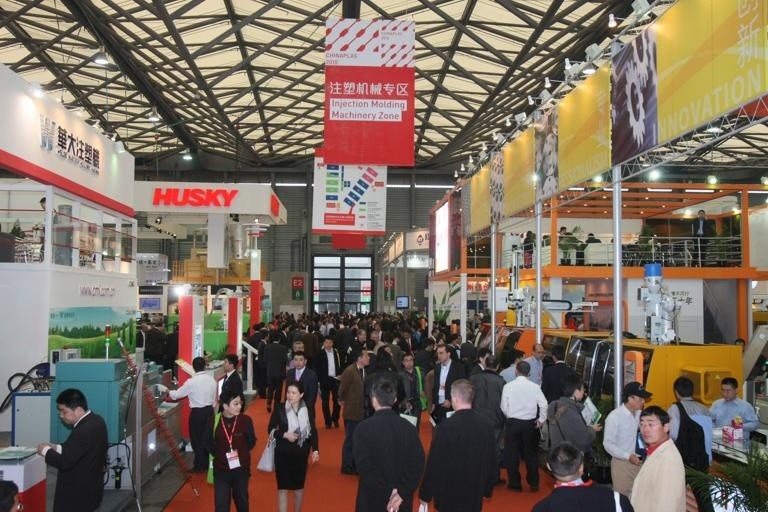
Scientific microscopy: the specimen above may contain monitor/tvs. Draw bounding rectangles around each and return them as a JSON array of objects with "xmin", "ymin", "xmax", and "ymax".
[{"xmin": 396, "ymin": 296, "xmax": 409, "ymax": 309}]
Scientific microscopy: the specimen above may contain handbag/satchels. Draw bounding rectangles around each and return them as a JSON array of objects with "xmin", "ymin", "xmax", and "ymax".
[
  {"xmin": 257, "ymin": 429, "xmax": 276, "ymax": 472},
  {"xmin": 675, "ymin": 421, "xmax": 709, "ymax": 471},
  {"xmin": 420, "ymin": 396, "xmax": 428, "ymax": 410},
  {"xmin": 399, "ymin": 408, "xmax": 418, "ymax": 427},
  {"xmin": 207, "ymin": 451, "xmax": 214, "ymax": 484}
]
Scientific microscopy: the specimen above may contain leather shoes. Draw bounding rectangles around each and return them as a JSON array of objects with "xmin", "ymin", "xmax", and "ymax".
[
  {"xmin": 326, "ymin": 415, "xmax": 339, "ymax": 428},
  {"xmin": 189, "ymin": 467, "xmax": 206, "ymax": 472},
  {"xmin": 508, "ymin": 480, "xmax": 538, "ymax": 491}
]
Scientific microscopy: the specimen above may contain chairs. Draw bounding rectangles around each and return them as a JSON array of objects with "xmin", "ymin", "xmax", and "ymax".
[{"xmin": 583, "ymin": 242, "xmax": 688, "ymax": 267}]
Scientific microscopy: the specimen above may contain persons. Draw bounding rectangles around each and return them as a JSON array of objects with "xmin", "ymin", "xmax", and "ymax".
[
  {"xmin": 268, "ymin": 382, "xmax": 319, "ymax": 511},
  {"xmin": 510, "ymin": 227, "xmax": 614, "ymax": 267},
  {"xmin": 135, "ymin": 311, "xmax": 180, "ymax": 370},
  {"xmin": 735, "ymin": 338, "xmax": 745, "ymax": 354},
  {"xmin": 690, "ymin": 209, "xmax": 715, "ymax": 266},
  {"xmin": 664, "ymin": 377, "xmax": 715, "ymax": 512},
  {"xmin": 502, "ymin": 362, "xmax": 549, "ymax": 491},
  {"xmin": 419, "ymin": 379, "xmax": 498, "ymax": 511},
  {"xmin": 601, "ymin": 382, "xmax": 654, "ymax": 497},
  {"xmin": 35, "ymin": 198, "xmax": 58, "ymax": 260},
  {"xmin": 242, "ymin": 311, "xmax": 581, "ymax": 482},
  {"xmin": 757, "ymin": 364, "xmax": 767, "ymax": 383},
  {"xmin": 629, "ymin": 406, "xmax": 687, "ymax": 512},
  {"xmin": 530, "ymin": 440, "xmax": 635, "ymax": 512},
  {"xmin": 210, "ymin": 390, "xmax": 255, "ymax": 510},
  {"xmin": 36, "ymin": 389, "xmax": 107, "ymax": 512},
  {"xmin": 0, "ymin": 481, "xmax": 19, "ymax": 512},
  {"xmin": 708, "ymin": 377, "xmax": 760, "ymax": 439},
  {"xmin": 340, "ymin": 380, "xmax": 424, "ymax": 512},
  {"xmin": 168, "ymin": 353, "xmax": 241, "ymax": 471},
  {"xmin": 547, "ymin": 373, "xmax": 602, "ymax": 453}
]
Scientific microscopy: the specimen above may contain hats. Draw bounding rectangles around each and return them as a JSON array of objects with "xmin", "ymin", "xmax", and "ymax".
[{"xmin": 624, "ymin": 382, "xmax": 652, "ymax": 398}]
